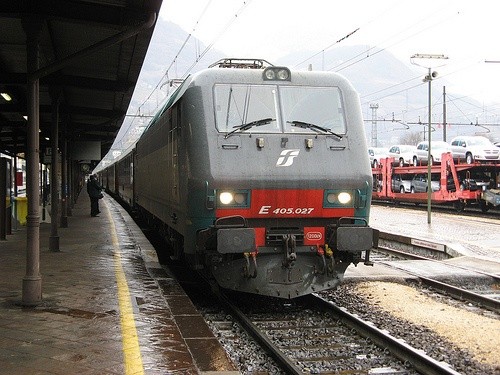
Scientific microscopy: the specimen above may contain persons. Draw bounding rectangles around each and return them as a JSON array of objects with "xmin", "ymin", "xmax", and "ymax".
[{"xmin": 87, "ymin": 173, "xmax": 104, "ymax": 217}]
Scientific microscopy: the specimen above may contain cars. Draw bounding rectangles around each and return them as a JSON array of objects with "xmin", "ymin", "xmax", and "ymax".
[{"xmin": 369, "ymin": 135, "xmax": 499, "ymax": 195}]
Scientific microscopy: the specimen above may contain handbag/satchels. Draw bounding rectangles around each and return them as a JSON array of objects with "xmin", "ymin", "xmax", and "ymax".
[{"xmin": 96, "ymin": 190, "xmax": 104, "ymax": 199}]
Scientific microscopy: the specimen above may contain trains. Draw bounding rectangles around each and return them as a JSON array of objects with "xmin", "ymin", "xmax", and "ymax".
[{"xmin": 94, "ymin": 59, "xmax": 381, "ymax": 304}]
